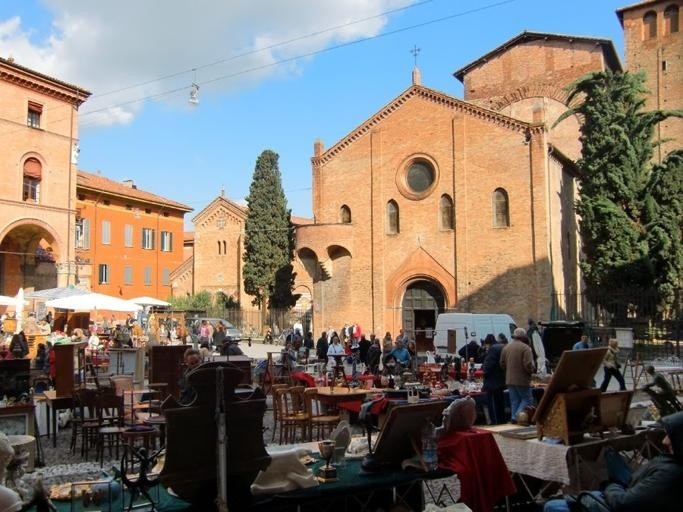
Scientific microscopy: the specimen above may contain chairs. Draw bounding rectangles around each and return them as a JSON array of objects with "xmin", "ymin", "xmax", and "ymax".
[{"xmin": 0, "ymin": 326, "xmax": 682, "ymax": 512}]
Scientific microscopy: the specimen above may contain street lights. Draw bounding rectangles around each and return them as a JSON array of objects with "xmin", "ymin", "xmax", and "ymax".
[{"xmin": 74, "ymin": 255, "xmax": 81, "ymax": 284}]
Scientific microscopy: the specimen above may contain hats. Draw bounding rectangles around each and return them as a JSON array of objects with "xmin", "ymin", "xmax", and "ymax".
[
  {"xmin": 511, "ymin": 328, "xmax": 529, "ymax": 339},
  {"xmin": 484, "ymin": 333, "xmax": 497, "ymax": 344}
]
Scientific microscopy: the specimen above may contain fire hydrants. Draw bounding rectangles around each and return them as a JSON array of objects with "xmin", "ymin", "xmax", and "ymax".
[{"xmin": 247, "ymin": 337, "xmax": 252, "ymax": 346}]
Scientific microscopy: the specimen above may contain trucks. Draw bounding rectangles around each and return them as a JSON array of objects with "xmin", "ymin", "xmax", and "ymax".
[{"xmin": 433, "ymin": 313, "xmax": 520, "ymax": 363}]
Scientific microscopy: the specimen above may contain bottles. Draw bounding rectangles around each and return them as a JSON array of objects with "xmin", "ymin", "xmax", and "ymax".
[
  {"xmin": 419, "ymin": 416, "xmax": 439, "ymax": 471},
  {"xmin": 326, "ymin": 350, "xmax": 485, "ymax": 396}
]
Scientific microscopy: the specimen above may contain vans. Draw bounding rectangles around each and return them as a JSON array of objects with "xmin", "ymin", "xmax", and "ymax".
[
  {"xmin": 184, "ymin": 315, "xmax": 242, "ymax": 346},
  {"xmin": 536, "ymin": 318, "xmax": 587, "ymax": 373}
]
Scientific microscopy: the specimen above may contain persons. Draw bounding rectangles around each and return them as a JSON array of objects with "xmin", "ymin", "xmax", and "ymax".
[
  {"xmin": 498, "ymin": 327, "xmax": 538, "ymax": 424},
  {"xmin": 640, "ymin": 362, "xmax": 677, "ymax": 407},
  {"xmin": 599, "ymin": 338, "xmax": 630, "ymax": 391},
  {"xmin": 540, "ymin": 407, "xmax": 683, "ymax": 512},
  {"xmin": 571, "ymin": 332, "xmax": 594, "ymax": 354}
]
